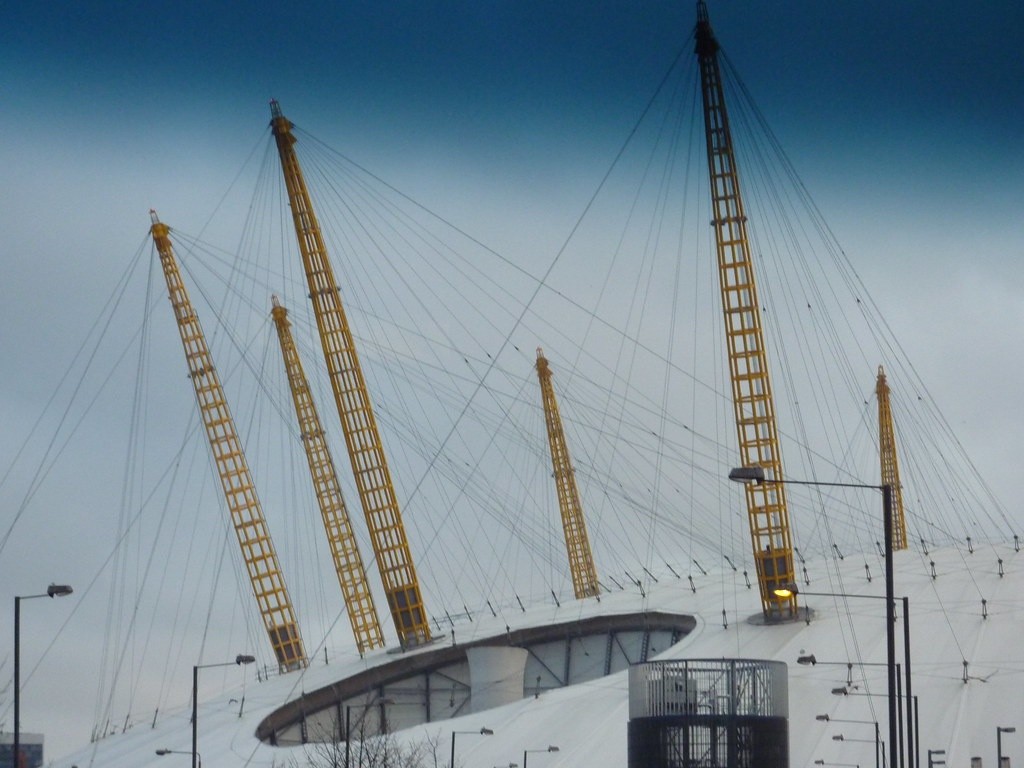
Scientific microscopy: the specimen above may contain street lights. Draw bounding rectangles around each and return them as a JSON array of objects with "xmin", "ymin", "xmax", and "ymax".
[
  {"xmin": 728, "ymin": 463, "xmax": 897, "ymax": 768},
  {"xmin": 832, "ymin": 733, "xmax": 886, "ymax": 768},
  {"xmin": 12, "ymin": 583, "xmax": 75, "ymax": 768},
  {"xmin": 794, "ymin": 653, "xmax": 906, "ymax": 768},
  {"xmin": 815, "ymin": 713, "xmax": 880, "ymax": 768},
  {"xmin": 155, "ymin": 748, "xmax": 202, "ymax": 768},
  {"xmin": 928, "ymin": 749, "xmax": 946, "ymax": 768},
  {"xmin": 770, "ymin": 582, "xmax": 915, "ymax": 768},
  {"xmin": 996, "ymin": 726, "xmax": 1018, "ymax": 768},
  {"xmin": 450, "ymin": 725, "xmax": 494, "ymax": 768},
  {"xmin": 830, "ymin": 687, "xmax": 921, "ymax": 768},
  {"xmin": 522, "ymin": 745, "xmax": 559, "ymax": 767},
  {"xmin": 191, "ymin": 654, "xmax": 257, "ymax": 768},
  {"xmin": 344, "ymin": 699, "xmax": 396, "ymax": 768}
]
